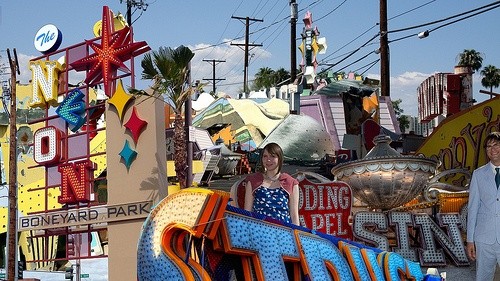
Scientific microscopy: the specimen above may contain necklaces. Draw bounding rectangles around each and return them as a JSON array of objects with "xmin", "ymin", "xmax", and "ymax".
[{"xmin": 265, "ymin": 171, "xmax": 280, "ymax": 182}]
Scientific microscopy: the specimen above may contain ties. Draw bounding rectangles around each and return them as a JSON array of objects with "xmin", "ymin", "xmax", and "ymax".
[{"xmin": 494, "ymin": 168, "xmax": 500, "ymax": 189}]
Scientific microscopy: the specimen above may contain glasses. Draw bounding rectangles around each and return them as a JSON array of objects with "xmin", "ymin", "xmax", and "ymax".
[{"xmin": 484, "ymin": 144, "xmax": 500, "ymax": 150}]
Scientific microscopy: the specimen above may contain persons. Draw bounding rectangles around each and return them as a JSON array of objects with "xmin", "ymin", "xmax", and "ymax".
[
  {"xmin": 466, "ymin": 132, "xmax": 500, "ymax": 281},
  {"xmin": 244, "ymin": 142, "xmax": 300, "ymax": 226}
]
